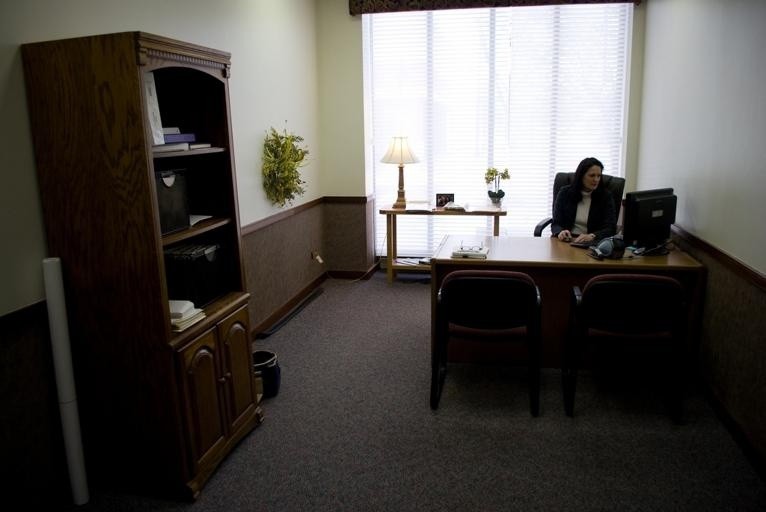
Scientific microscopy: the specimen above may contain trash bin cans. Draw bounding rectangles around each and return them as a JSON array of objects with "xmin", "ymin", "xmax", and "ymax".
[{"xmin": 252, "ymin": 350, "xmax": 280, "ymax": 399}]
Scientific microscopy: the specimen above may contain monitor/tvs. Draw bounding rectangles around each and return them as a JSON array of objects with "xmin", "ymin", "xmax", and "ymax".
[{"xmin": 623, "ymin": 188, "xmax": 677, "ymax": 256}]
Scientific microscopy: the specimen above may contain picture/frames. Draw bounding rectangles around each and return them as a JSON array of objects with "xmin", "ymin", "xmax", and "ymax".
[{"xmin": 436, "ymin": 193, "xmax": 454, "ymax": 207}]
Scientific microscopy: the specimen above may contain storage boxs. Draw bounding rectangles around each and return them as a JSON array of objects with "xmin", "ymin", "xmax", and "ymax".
[
  {"xmin": 163, "ymin": 241, "xmax": 226, "ymax": 311},
  {"xmin": 154, "ymin": 166, "xmax": 192, "ymax": 239}
]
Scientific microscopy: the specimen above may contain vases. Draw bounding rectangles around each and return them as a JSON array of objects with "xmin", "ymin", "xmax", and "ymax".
[{"xmin": 489, "ymin": 197, "xmax": 500, "ymax": 203}]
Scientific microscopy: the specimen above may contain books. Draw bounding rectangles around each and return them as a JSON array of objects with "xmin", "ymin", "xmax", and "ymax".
[
  {"xmin": 166, "ymin": 294, "xmax": 207, "ymax": 335},
  {"xmin": 145, "ymin": 70, "xmax": 211, "ymax": 151},
  {"xmin": 444, "ymin": 201, "xmax": 467, "ymax": 211},
  {"xmin": 395, "ymin": 256, "xmax": 431, "ymax": 265}
]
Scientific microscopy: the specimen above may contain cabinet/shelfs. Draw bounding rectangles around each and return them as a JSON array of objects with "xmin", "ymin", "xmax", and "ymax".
[{"xmin": 18, "ymin": 30, "xmax": 263, "ymax": 504}]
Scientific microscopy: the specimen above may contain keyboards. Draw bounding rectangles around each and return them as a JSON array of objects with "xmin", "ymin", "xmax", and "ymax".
[{"xmin": 570, "ymin": 239, "xmax": 602, "ymax": 247}]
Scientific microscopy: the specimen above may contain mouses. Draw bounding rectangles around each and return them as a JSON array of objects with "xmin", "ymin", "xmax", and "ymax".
[{"xmin": 563, "ymin": 235, "xmax": 573, "ymax": 242}]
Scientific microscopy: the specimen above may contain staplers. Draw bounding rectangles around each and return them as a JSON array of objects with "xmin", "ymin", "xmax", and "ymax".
[{"xmin": 587, "ymin": 246, "xmax": 604, "ymax": 260}]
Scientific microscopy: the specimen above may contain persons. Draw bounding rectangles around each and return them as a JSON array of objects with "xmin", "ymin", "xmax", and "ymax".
[{"xmin": 550, "ymin": 157, "xmax": 619, "ymax": 244}]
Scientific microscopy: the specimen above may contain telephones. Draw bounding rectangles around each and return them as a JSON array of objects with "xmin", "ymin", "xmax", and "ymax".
[{"xmin": 443, "ymin": 201, "xmax": 466, "ymax": 210}]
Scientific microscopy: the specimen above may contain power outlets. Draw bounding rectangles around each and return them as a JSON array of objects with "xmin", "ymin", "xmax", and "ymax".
[{"xmin": 311, "ymin": 248, "xmax": 321, "ymax": 264}]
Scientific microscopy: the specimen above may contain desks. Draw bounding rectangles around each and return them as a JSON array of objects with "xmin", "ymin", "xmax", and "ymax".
[
  {"xmin": 378, "ymin": 206, "xmax": 507, "ymax": 285},
  {"xmin": 430, "ymin": 235, "xmax": 705, "ymax": 369}
]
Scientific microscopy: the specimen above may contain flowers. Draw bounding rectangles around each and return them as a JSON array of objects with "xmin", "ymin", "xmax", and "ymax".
[{"xmin": 485, "ymin": 167, "xmax": 510, "ymax": 198}]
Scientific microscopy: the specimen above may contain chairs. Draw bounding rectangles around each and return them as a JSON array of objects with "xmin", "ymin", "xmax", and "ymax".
[
  {"xmin": 428, "ymin": 268, "xmax": 543, "ymax": 419},
  {"xmin": 534, "ymin": 172, "xmax": 626, "ymax": 237},
  {"xmin": 560, "ymin": 271, "xmax": 701, "ymax": 423}
]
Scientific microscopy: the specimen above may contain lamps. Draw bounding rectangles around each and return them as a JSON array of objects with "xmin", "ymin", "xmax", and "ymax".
[{"xmin": 378, "ymin": 135, "xmax": 419, "ymax": 210}]
